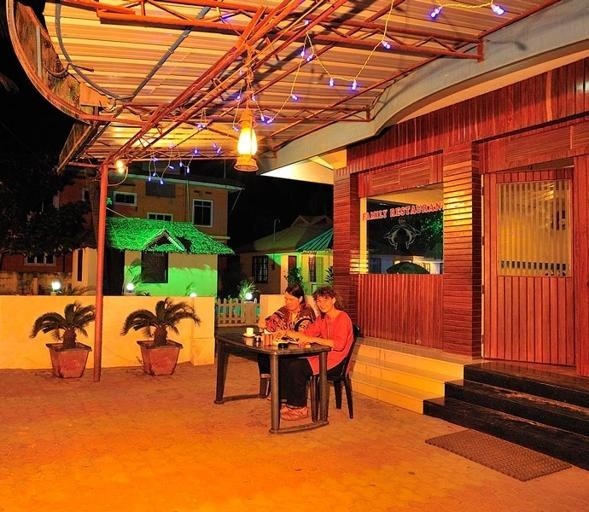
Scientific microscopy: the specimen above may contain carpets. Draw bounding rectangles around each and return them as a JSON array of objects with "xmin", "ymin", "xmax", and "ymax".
[{"xmin": 424, "ymin": 425, "xmax": 571, "ymax": 483}]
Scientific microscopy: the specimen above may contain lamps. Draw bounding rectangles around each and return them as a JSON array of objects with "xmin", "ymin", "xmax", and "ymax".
[{"xmin": 233, "ymin": 77, "xmax": 260, "ymax": 173}]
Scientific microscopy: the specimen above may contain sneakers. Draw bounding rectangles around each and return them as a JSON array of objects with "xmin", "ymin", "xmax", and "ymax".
[{"xmin": 281, "ymin": 403, "xmax": 311, "ymax": 421}]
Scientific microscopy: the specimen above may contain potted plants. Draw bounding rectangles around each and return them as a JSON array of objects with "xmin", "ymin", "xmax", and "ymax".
[
  {"xmin": 27, "ymin": 300, "xmax": 95, "ymax": 377},
  {"xmin": 120, "ymin": 296, "xmax": 203, "ymax": 374}
]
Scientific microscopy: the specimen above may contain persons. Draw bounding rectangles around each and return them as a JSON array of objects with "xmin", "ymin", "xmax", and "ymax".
[
  {"xmin": 277, "ymin": 283, "xmax": 354, "ymax": 422},
  {"xmin": 257, "ymin": 282, "xmax": 316, "ymax": 409}
]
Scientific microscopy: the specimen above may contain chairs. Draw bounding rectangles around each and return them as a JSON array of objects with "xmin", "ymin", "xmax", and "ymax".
[{"xmin": 310, "ymin": 324, "xmax": 363, "ymax": 419}]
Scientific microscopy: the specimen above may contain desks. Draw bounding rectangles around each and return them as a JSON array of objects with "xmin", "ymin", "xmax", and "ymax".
[{"xmin": 214, "ymin": 325, "xmax": 332, "ymax": 435}]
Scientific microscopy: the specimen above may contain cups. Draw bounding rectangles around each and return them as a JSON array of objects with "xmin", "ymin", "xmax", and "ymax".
[{"xmin": 247, "ymin": 327, "xmax": 260, "ymax": 341}]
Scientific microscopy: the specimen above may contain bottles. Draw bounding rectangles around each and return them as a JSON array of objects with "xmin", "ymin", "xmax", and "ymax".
[{"xmin": 261, "ymin": 332, "xmax": 272, "ymax": 346}]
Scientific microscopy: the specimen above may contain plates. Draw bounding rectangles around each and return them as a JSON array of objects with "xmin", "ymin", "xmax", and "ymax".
[
  {"xmin": 243, "ymin": 333, "xmax": 256, "ymax": 337},
  {"xmin": 271, "ymin": 338, "xmax": 299, "ymax": 345}
]
